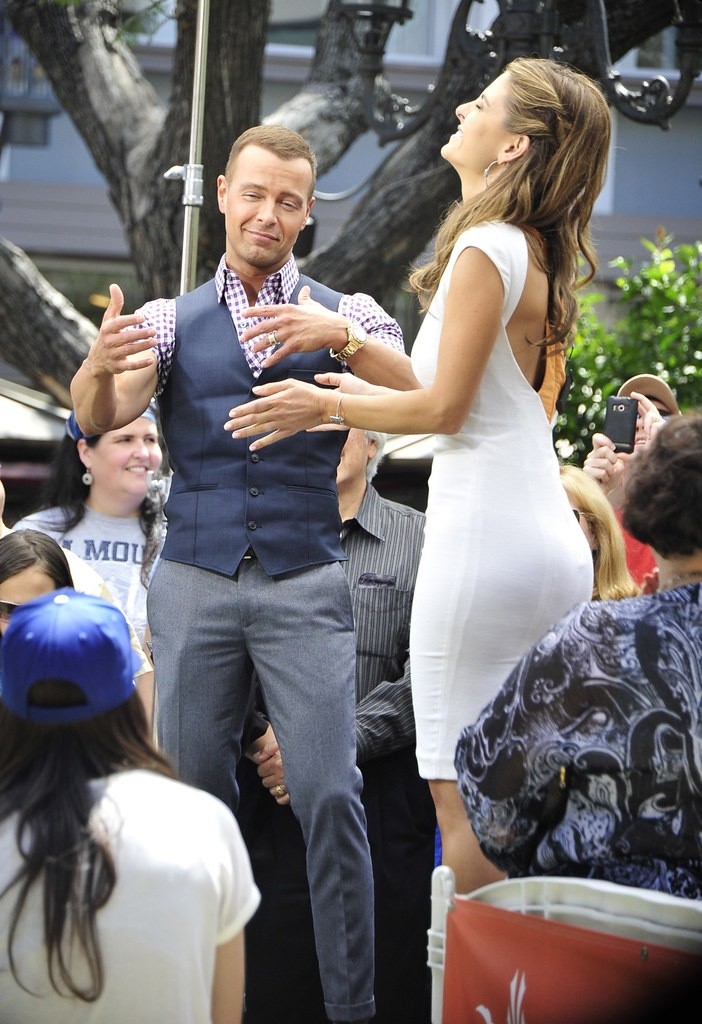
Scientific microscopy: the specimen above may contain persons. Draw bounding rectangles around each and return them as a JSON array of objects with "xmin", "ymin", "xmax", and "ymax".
[
  {"xmin": 455, "ymin": 374, "xmax": 702, "ymax": 902},
  {"xmin": 70, "ymin": 125, "xmax": 406, "ymax": 1024},
  {"xmin": 223, "ymin": 56, "xmax": 612, "ymax": 896},
  {"xmin": 13, "ymin": 404, "xmax": 169, "ymax": 665},
  {"xmin": 0, "ymin": 529, "xmax": 263, "ymax": 1023},
  {"xmin": 240, "ymin": 426, "xmax": 427, "ymax": 1024}
]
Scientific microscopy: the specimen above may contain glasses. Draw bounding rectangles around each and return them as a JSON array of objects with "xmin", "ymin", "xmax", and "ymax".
[
  {"xmin": 0, "ymin": 601, "xmax": 21, "ymax": 624},
  {"xmin": 636, "ymin": 408, "xmax": 675, "ymax": 421},
  {"xmin": 573, "ymin": 509, "xmax": 590, "ymax": 523}
]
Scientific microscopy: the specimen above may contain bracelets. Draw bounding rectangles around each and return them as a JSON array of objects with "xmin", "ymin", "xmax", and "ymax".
[{"xmin": 330, "ymin": 393, "xmax": 345, "ymax": 425}]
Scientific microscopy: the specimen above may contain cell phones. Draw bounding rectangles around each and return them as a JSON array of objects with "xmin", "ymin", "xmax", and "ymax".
[{"xmin": 603, "ymin": 396, "xmax": 639, "ymax": 455}]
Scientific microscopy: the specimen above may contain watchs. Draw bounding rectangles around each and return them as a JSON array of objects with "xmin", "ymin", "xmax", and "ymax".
[{"xmin": 330, "ymin": 319, "xmax": 369, "ymax": 362}]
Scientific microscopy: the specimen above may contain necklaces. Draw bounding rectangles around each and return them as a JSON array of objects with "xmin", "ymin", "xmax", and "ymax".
[{"xmin": 657, "ymin": 568, "xmax": 701, "ymax": 592}]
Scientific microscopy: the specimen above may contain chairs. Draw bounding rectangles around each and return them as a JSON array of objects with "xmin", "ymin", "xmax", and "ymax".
[{"xmin": 428, "ymin": 864, "xmax": 702, "ymax": 1024}]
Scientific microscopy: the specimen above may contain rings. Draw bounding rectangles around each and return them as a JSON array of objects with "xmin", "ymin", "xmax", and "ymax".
[{"xmin": 275, "ymin": 785, "xmax": 284, "ymax": 795}]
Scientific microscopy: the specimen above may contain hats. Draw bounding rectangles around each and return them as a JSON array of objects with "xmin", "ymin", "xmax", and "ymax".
[
  {"xmin": 616, "ymin": 374, "xmax": 683, "ymax": 417},
  {"xmin": 0, "ymin": 585, "xmax": 138, "ymax": 726}
]
